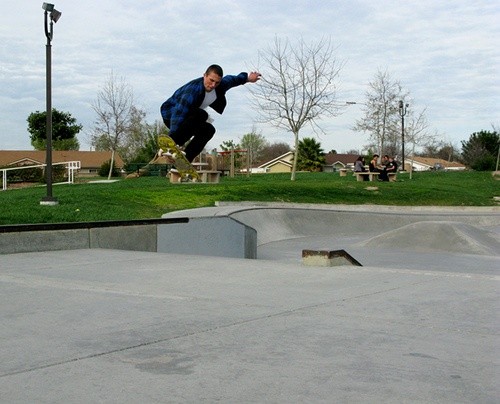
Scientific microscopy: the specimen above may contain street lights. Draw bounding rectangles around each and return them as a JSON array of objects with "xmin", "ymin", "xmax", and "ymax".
[
  {"xmin": 398, "ymin": 100, "xmax": 409, "ymax": 170},
  {"xmin": 40, "ymin": 2, "xmax": 62, "ymax": 206}
]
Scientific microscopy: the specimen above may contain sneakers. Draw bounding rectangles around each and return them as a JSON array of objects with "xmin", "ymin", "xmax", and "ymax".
[
  {"xmin": 177, "ymin": 145, "xmax": 186, "ymax": 156},
  {"xmin": 170, "ymin": 166, "xmax": 178, "ymax": 173}
]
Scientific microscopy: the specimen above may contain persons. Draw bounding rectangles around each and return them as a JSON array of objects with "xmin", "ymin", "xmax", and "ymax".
[
  {"xmin": 157, "ymin": 64, "xmax": 262, "ymax": 174},
  {"xmin": 354, "ymin": 154, "xmax": 398, "ymax": 182}
]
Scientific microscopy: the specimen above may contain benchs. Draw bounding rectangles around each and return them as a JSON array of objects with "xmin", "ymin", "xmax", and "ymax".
[
  {"xmin": 171, "ymin": 169, "xmax": 222, "ymax": 182},
  {"xmin": 339, "ymin": 169, "xmax": 349, "ymax": 177},
  {"xmin": 355, "ymin": 172, "xmax": 397, "ymax": 182}
]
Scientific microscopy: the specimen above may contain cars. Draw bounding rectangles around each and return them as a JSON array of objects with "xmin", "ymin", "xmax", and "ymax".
[{"xmin": 429, "ymin": 163, "xmax": 446, "ymax": 172}]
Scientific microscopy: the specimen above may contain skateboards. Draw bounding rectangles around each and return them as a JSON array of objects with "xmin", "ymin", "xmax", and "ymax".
[{"xmin": 156, "ymin": 134, "xmax": 202, "ymax": 183}]
